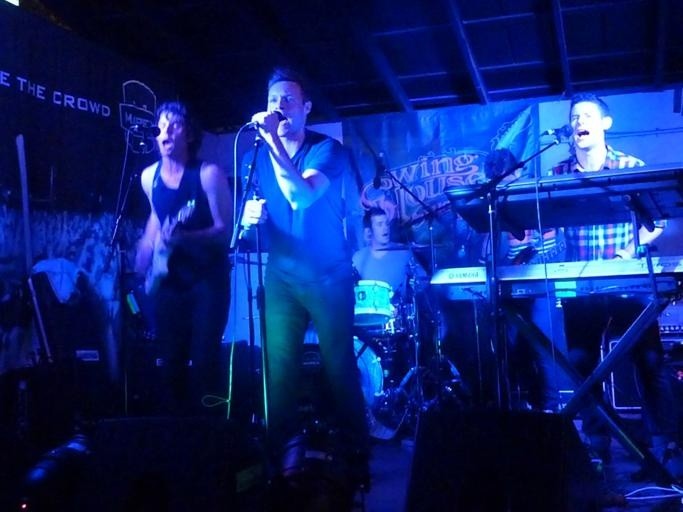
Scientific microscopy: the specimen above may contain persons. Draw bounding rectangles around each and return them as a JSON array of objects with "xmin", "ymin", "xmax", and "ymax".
[
  {"xmin": 543, "ymin": 90, "xmax": 683, "ymax": 484},
  {"xmin": 350, "ymin": 206, "xmax": 430, "ymax": 296},
  {"xmin": 237, "ymin": 67, "xmax": 374, "ymax": 492},
  {"xmin": 133, "ymin": 101, "xmax": 235, "ymax": 417},
  {"xmin": 483, "ymin": 147, "xmax": 564, "ymax": 413}
]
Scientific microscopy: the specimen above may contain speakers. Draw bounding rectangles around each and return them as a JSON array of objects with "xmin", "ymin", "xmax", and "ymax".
[{"xmin": 405, "ymin": 406, "xmax": 573, "ymax": 510}]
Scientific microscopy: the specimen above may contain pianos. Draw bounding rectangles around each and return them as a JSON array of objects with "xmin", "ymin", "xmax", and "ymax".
[
  {"xmin": 430, "ymin": 255, "xmax": 683, "ymax": 310},
  {"xmin": 444, "ymin": 166, "xmax": 683, "ymax": 233}
]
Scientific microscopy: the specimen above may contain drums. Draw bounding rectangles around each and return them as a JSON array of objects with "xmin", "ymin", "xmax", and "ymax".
[
  {"xmin": 367, "ymin": 298, "xmax": 407, "ymax": 344},
  {"xmin": 350, "ymin": 279, "xmax": 396, "ymax": 328}
]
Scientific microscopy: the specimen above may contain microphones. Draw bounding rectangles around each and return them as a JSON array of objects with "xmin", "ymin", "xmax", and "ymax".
[
  {"xmin": 133, "ymin": 125, "xmax": 161, "ymax": 138},
  {"xmin": 373, "ymin": 153, "xmax": 384, "ymax": 189},
  {"xmin": 545, "ymin": 125, "xmax": 574, "ymax": 137},
  {"xmin": 246, "ymin": 110, "xmax": 284, "ymax": 128}
]
